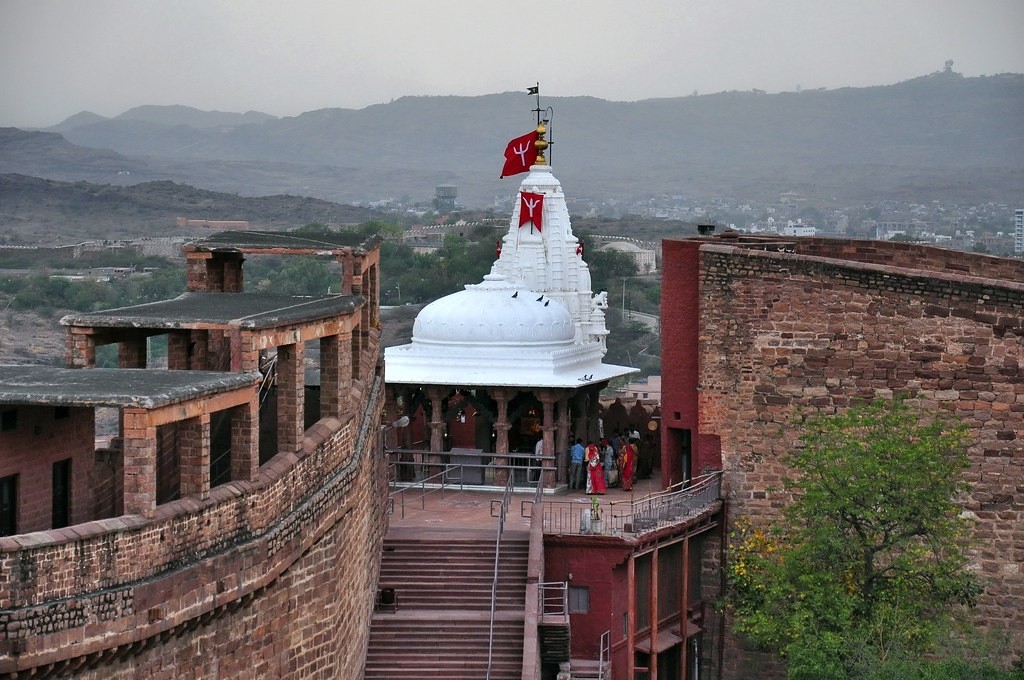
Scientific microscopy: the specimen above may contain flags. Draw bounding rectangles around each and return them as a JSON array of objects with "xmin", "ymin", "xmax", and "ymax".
[
  {"xmin": 519, "ymin": 192, "xmax": 543, "ymax": 233},
  {"xmin": 576, "ymin": 242, "xmax": 582, "ymax": 255},
  {"xmin": 496, "ymin": 241, "xmax": 501, "ymax": 256},
  {"xmin": 501, "ymin": 129, "xmax": 539, "ymax": 176}
]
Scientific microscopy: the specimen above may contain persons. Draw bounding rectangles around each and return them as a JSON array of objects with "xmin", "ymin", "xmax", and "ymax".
[
  {"xmin": 600, "ymin": 427, "xmax": 641, "ymax": 492},
  {"xmin": 585, "ymin": 441, "xmax": 607, "ymax": 495},
  {"xmin": 533, "ymin": 436, "xmax": 543, "ymax": 484},
  {"xmin": 568, "ymin": 437, "xmax": 584, "ymax": 491}
]
